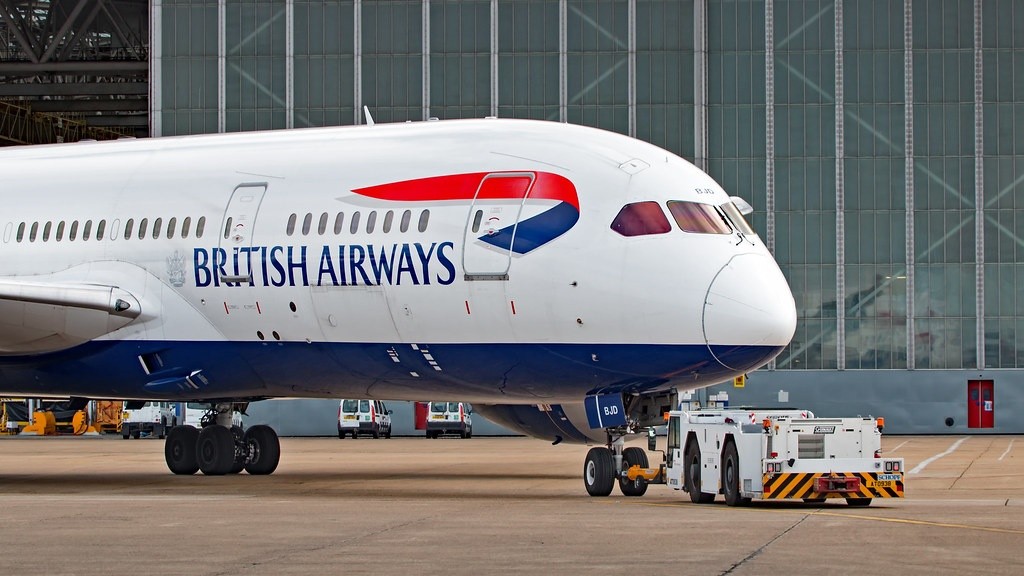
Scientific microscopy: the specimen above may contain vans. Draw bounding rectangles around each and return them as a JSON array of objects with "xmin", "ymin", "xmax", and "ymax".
[
  {"xmin": 423, "ymin": 401, "xmax": 472, "ymax": 439},
  {"xmin": 0, "ymin": 395, "xmax": 245, "ymax": 440},
  {"xmin": 337, "ymin": 398, "xmax": 393, "ymax": 439}
]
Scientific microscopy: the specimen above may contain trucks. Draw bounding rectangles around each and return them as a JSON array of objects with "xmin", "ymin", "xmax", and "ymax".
[{"xmin": 647, "ymin": 404, "xmax": 905, "ymax": 508}]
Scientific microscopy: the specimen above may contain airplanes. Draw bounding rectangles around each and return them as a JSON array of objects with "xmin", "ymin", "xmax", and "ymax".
[{"xmin": 0, "ymin": 117, "xmax": 795, "ymax": 497}]
